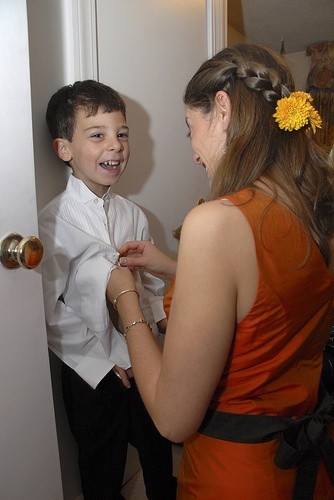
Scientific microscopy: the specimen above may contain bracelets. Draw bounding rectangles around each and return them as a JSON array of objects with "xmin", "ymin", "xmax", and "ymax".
[
  {"xmin": 113, "ymin": 289, "xmax": 140, "ymax": 311},
  {"xmin": 123, "ymin": 318, "xmax": 153, "ymax": 344}
]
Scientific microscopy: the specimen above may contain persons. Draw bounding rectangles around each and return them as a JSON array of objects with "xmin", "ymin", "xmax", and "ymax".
[
  {"xmin": 36, "ymin": 80, "xmax": 179, "ymax": 500},
  {"xmin": 104, "ymin": 43, "xmax": 333, "ymax": 499}
]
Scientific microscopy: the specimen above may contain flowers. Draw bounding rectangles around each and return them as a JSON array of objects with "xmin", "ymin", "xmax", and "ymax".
[{"xmin": 270, "ymin": 91, "xmax": 324, "ymax": 137}]
